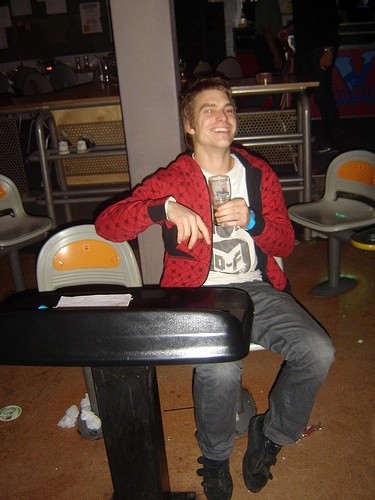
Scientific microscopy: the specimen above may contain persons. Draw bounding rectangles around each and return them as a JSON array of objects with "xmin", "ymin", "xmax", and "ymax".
[
  {"xmin": 277, "ymin": 0, "xmax": 341, "ymax": 153},
  {"xmin": 250, "ymin": 0, "xmax": 293, "ymax": 77},
  {"xmin": 94, "ymin": 78, "xmax": 334, "ymax": 500}
]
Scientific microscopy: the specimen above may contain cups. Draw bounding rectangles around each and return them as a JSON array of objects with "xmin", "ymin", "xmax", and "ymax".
[
  {"xmin": 58, "ymin": 140, "xmax": 69, "ymax": 155},
  {"xmin": 77, "ymin": 140, "xmax": 87, "ymax": 154},
  {"xmin": 256, "ymin": 73, "xmax": 273, "ymax": 85},
  {"xmin": 209, "ymin": 175, "xmax": 231, "ymax": 226}
]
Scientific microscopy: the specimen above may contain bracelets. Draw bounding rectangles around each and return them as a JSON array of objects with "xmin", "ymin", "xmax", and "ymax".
[
  {"xmin": 245, "ymin": 208, "xmax": 256, "ymax": 230},
  {"xmin": 323, "ymin": 48, "xmax": 334, "ymax": 52}
]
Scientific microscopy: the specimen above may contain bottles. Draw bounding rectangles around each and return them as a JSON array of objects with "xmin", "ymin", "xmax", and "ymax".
[
  {"xmin": 104, "ymin": 64, "xmax": 111, "ymax": 88},
  {"xmin": 281, "ymin": 34, "xmax": 290, "ymax": 63}
]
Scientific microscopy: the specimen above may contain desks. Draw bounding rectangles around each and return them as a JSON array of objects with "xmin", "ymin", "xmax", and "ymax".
[{"xmin": 0, "ymin": 69, "xmax": 322, "ymax": 242}]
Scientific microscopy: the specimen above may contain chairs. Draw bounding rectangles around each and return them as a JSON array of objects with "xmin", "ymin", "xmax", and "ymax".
[
  {"xmin": 235, "ymin": 256, "xmax": 284, "ymax": 437},
  {"xmin": 36, "ymin": 225, "xmax": 143, "ymax": 441},
  {"xmin": 286, "ymin": 149, "xmax": 375, "ymax": 297},
  {"xmin": 0, "ymin": 173, "xmax": 53, "ymax": 292}
]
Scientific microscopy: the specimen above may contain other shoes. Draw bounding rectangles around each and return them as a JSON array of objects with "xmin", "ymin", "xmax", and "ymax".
[
  {"xmin": 196, "ymin": 456, "xmax": 233, "ymax": 500},
  {"xmin": 243, "ymin": 414, "xmax": 283, "ymax": 493}
]
